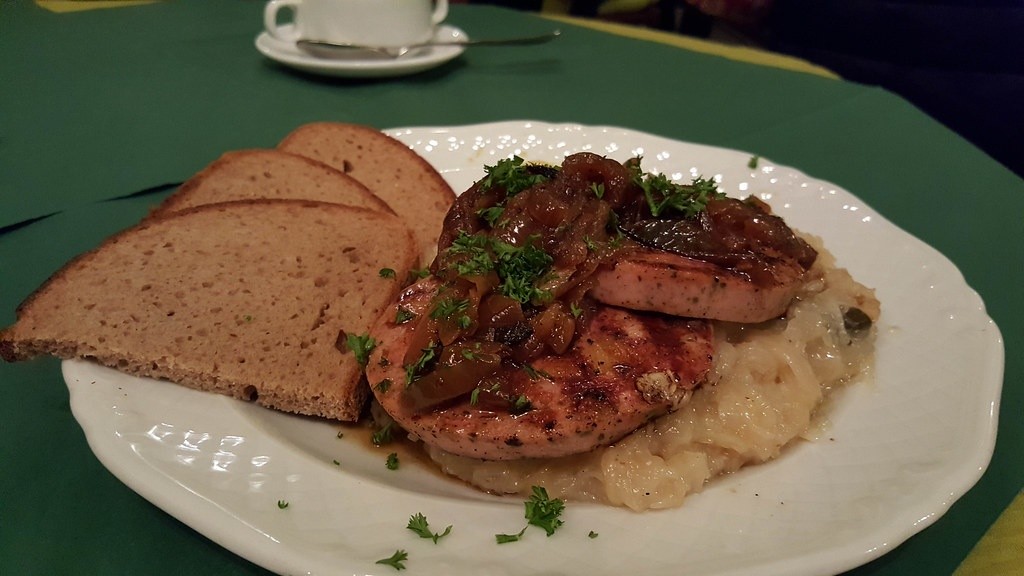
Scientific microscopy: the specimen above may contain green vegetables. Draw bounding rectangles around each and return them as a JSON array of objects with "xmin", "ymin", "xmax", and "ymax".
[{"xmin": 332, "ymin": 152, "xmax": 729, "ymax": 569}]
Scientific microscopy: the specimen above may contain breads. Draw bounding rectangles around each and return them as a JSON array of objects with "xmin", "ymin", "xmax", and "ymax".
[
  {"xmin": 148, "ymin": 148, "xmax": 421, "ymax": 285},
  {"xmin": 275, "ymin": 122, "xmax": 458, "ymax": 275},
  {"xmin": 0, "ymin": 197, "xmax": 423, "ymax": 424}
]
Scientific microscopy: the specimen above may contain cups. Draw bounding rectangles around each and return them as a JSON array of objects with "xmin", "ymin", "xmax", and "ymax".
[{"xmin": 263, "ymin": 0, "xmax": 448, "ymax": 59}]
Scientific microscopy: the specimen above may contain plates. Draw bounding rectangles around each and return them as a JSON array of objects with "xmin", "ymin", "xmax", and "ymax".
[
  {"xmin": 60, "ymin": 118, "xmax": 1006, "ymax": 576},
  {"xmin": 254, "ymin": 21, "xmax": 470, "ymax": 78}
]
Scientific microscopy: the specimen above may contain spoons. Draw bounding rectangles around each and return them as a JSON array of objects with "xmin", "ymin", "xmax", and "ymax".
[{"xmin": 296, "ymin": 30, "xmax": 564, "ymax": 60}]
